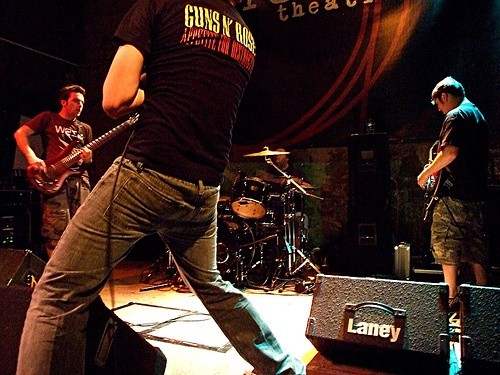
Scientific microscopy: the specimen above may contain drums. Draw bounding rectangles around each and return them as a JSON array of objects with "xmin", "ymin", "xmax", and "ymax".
[
  {"xmin": 214, "ymin": 216, "xmax": 257, "ymax": 275},
  {"xmin": 261, "ymin": 193, "xmax": 283, "ymax": 228},
  {"xmin": 230, "ymin": 175, "xmax": 272, "ymax": 220}
]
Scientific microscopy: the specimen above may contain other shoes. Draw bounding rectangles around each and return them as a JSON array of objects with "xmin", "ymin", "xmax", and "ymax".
[{"xmin": 449, "ymin": 296, "xmax": 459, "ymax": 312}]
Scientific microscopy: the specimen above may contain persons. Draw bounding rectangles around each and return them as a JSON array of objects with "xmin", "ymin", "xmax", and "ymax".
[
  {"xmin": 251, "ymin": 147, "xmax": 313, "ymax": 249},
  {"xmin": 15, "ymin": 86, "xmax": 93, "ymax": 259},
  {"xmin": 417, "ymin": 76, "xmax": 491, "ymax": 309},
  {"xmin": 17, "ymin": 0, "xmax": 306, "ymax": 375}
]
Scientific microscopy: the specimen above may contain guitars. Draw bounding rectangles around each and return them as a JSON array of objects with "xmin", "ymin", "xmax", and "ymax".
[
  {"xmin": 28, "ymin": 112, "xmax": 139, "ymax": 194},
  {"xmin": 424, "ymin": 144, "xmax": 455, "ymax": 224}
]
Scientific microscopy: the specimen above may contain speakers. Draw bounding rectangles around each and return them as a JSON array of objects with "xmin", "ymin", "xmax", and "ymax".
[
  {"xmin": 458, "ymin": 286, "xmax": 500, "ymax": 365},
  {"xmin": 305, "ymin": 274, "xmax": 450, "ymax": 367},
  {"xmin": 1, "ymin": 249, "xmax": 168, "ymax": 374}
]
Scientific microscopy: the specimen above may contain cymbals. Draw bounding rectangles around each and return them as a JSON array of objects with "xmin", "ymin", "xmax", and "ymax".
[
  {"xmin": 300, "ymin": 184, "xmax": 320, "ymax": 189},
  {"xmin": 243, "ymin": 150, "xmax": 291, "ymax": 156}
]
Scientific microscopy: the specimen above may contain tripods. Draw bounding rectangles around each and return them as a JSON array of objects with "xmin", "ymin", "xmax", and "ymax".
[{"xmin": 268, "ymin": 161, "xmax": 324, "ymax": 292}]
